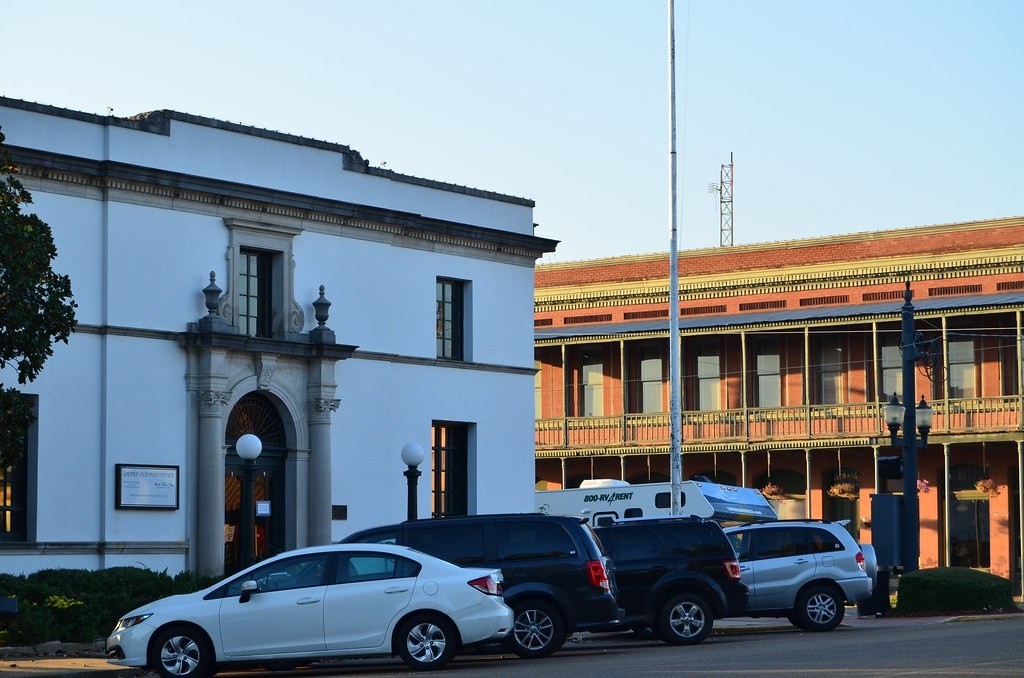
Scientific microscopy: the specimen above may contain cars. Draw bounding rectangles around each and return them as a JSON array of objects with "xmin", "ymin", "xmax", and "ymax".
[{"xmin": 106, "ymin": 543, "xmax": 516, "ymax": 678}]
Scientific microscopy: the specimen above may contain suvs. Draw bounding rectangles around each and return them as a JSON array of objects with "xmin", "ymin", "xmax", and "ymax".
[
  {"xmin": 723, "ymin": 517, "xmax": 878, "ymax": 633},
  {"xmin": 589, "ymin": 514, "xmax": 753, "ymax": 645},
  {"xmin": 271, "ymin": 511, "xmax": 626, "ymax": 658}
]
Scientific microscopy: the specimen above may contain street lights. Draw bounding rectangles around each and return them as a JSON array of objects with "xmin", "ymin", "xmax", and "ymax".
[
  {"xmin": 401, "ymin": 443, "xmax": 427, "ymax": 520},
  {"xmin": 236, "ymin": 432, "xmax": 263, "ymax": 571},
  {"xmin": 882, "ymin": 279, "xmax": 935, "ymax": 570}
]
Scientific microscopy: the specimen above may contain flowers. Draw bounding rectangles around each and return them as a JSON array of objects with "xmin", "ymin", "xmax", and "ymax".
[
  {"xmin": 826, "ymin": 471, "xmax": 864, "ymax": 496},
  {"xmin": 916, "ymin": 479, "xmax": 930, "ymax": 494},
  {"xmin": 974, "ymin": 478, "xmax": 1004, "ymax": 497},
  {"xmin": 762, "ymin": 483, "xmax": 786, "ymax": 497}
]
